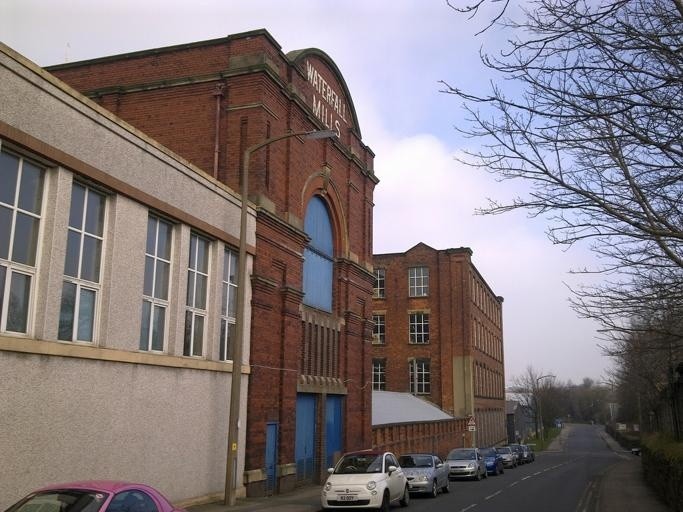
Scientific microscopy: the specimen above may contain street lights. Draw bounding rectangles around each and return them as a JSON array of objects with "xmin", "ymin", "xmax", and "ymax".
[
  {"xmin": 534, "ymin": 374, "xmax": 557, "ymax": 440},
  {"xmin": 221, "ymin": 128, "xmax": 339, "ymax": 506}
]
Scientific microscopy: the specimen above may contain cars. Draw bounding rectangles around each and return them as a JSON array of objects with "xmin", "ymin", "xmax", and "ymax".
[
  {"xmin": 321, "ymin": 449, "xmax": 411, "ymax": 512},
  {"xmin": 496, "ymin": 446, "xmax": 516, "ymax": 468},
  {"xmin": 519, "ymin": 444, "xmax": 536, "ymax": 463},
  {"xmin": 477, "ymin": 446, "xmax": 505, "ymax": 475},
  {"xmin": 507, "ymin": 443, "xmax": 523, "ymax": 465},
  {"xmin": 444, "ymin": 446, "xmax": 487, "ymax": 482},
  {"xmin": 398, "ymin": 451, "xmax": 452, "ymax": 497},
  {"xmin": 1, "ymin": 480, "xmax": 185, "ymax": 512}
]
